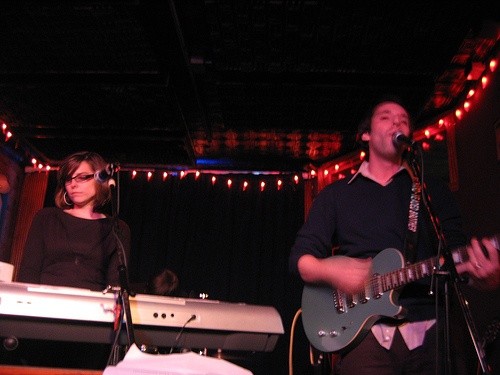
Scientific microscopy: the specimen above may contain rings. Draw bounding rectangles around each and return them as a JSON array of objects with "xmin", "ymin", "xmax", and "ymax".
[{"xmin": 474, "ymin": 263, "xmax": 481, "ymax": 270}]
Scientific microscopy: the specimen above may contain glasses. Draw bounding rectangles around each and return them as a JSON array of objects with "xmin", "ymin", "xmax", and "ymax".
[{"xmin": 64, "ymin": 172, "xmax": 94, "ymax": 186}]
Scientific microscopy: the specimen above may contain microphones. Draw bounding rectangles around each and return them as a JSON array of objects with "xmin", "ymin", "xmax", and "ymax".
[
  {"xmin": 392, "ymin": 130, "xmax": 420, "ymax": 151},
  {"xmin": 93, "ymin": 159, "xmax": 122, "ymax": 184}
]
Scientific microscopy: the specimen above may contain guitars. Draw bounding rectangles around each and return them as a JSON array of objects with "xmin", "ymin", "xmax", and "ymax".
[{"xmin": 302, "ymin": 235, "xmax": 500, "ymax": 355}]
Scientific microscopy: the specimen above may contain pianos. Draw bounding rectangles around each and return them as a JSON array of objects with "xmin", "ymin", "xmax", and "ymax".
[{"xmin": 0, "ymin": 281, "xmax": 285, "ymax": 354}]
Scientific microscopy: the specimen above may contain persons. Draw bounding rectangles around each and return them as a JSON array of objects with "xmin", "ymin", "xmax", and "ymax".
[
  {"xmin": 15, "ymin": 151, "xmax": 130, "ymax": 371},
  {"xmin": 288, "ymin": 96, "xmax": 500, "ymax": 375}
]
